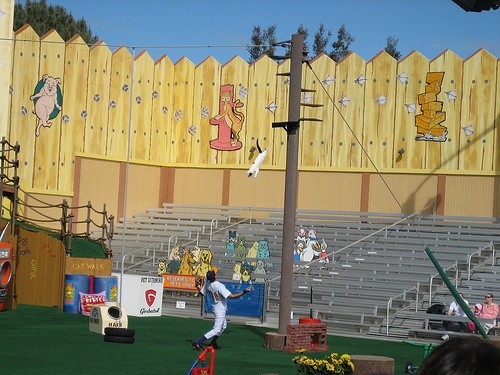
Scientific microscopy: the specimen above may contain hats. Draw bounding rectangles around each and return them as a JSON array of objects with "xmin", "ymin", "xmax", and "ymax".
[{"xmin": 475, "ymin": 304, "xmax": 482, "ymax": 310}]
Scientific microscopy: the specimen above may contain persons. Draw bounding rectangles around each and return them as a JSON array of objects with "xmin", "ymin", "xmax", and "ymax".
[
  {"xmin": 472, "ymin": 293, "xmax": 500, "ymax": 334},
  {"xmin": 193, "ymin": 270, "xmax": 252, "ymax": 350},
  {"xmin": 441, "ymin": 293, "xmax": 468, "ymax": 341}
]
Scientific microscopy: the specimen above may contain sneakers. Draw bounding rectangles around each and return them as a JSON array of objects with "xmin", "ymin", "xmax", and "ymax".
[{"xmin": 441, "ymin": 334, "xmax": 449, "ymax": 340}]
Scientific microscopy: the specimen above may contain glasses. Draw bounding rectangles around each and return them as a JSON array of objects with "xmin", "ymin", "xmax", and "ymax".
[{"xmin": 484, "ymin": 296, "xmax": 492, "ymax": 298}]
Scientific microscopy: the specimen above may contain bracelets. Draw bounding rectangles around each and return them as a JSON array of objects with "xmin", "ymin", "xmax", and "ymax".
[{"xmin": 243, "ymin": 290, "xmax": 247, "ymax": 294}]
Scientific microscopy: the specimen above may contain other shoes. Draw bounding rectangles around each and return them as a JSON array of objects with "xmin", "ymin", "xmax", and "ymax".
[
  {"xmin": 211, "ymin": 345, "xmax": 222, "ymax": 350},
  {"xmin": 192, "ymin": 343, "xmax": 204, "ymax": 352}
]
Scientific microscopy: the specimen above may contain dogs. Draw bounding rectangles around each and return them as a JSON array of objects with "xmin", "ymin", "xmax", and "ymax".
[{"xmin": 247, "ymin": 139, "xmax": 269, "ymax": 178}]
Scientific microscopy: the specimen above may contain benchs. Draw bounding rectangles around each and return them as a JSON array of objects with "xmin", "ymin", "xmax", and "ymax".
[{"xmin": 88, "ymin": 202, "xmax": 500, "ymax": 340}]
